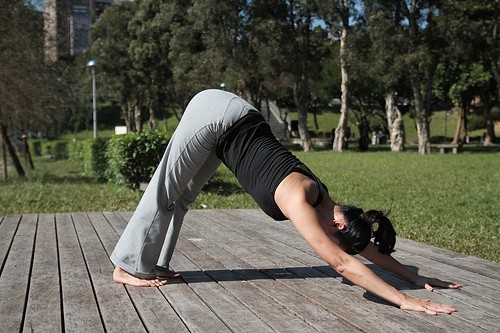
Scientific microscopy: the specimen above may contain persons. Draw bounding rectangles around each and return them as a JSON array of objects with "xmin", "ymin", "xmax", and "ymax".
[
  {"xmin": 484, "ymin": 132, "xmax": 496, "ymax": 145},
  {"xmin": 481, "ymin": 132, "xmax": 487, "ymax": 144},
  {"xmin": 357, "ymin": 120, "xmax": 369, "ymax": 150},
  {"xmin": 376, "ymin": 128, "xmax": 386, "ymax": 144},
  {"xmin": 109, "ymin": 88, "xmax": 463, "ymax": 316}
]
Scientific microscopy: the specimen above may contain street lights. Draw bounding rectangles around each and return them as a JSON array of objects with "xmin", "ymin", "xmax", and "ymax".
[{"xmin": 86, "ymin": 61, "xmax": 99, "ymax": 141}]
